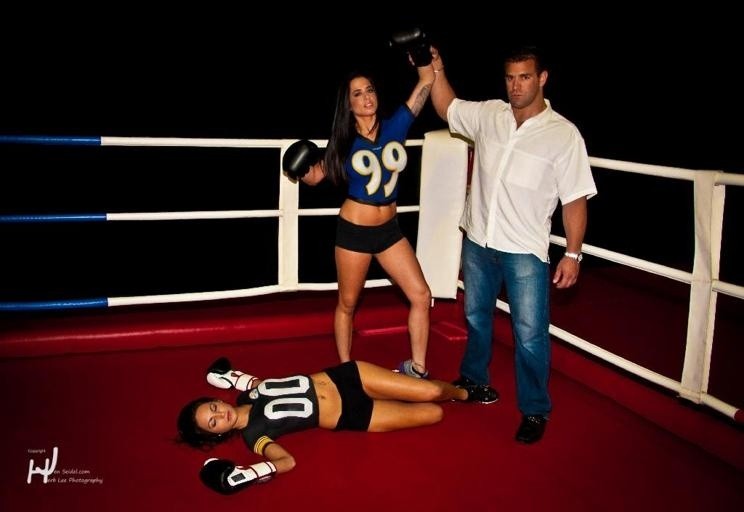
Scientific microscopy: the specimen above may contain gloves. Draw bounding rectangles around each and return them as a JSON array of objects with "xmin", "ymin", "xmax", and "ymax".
[
  {"xmin": 199, "ymin": 457, "xmax": 277, "ymax": 495},
  {"xmin": 389, "ymin": 23, "xmax": 433, "ymax": 68},
  {"xmin": 206, "ymin": 357, "xmax": 258, "ymax": 392},
  {"xmin": 282, "ymin": 140, "xmax": 323, "ymax": 181}
]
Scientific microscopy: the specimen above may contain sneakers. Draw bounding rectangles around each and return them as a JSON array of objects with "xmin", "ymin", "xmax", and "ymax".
[
  {"xmin": 513, "ymin": 414, "xmax": 550, "ymax": 444},
  {"xmin": 448, "ymin": 377, "xmax": 499, "ymax": 404},
  {"xmin": 399, "ymin": 358, "xmax": 429, "ymax": 380}
]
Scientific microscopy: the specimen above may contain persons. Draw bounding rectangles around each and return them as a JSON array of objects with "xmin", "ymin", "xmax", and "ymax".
[
  {"xmin": 172, "ymin": 355, "xmax": 500, "ymax": 495},
  {"xmin": 281, "ymin": 9, "xmax": 438, "ymax": 380},
  {"xmin": 401, "ymin": 45, "xmax": 601, "ymax": 444}
]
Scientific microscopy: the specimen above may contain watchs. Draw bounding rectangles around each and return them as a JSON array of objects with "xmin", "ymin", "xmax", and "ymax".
[{"xmin": 563, "ymin": 251, "xmax": 583, "ymax": 264}]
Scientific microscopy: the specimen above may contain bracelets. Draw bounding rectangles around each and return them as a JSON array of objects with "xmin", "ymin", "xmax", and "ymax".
[{"xmin": 431, "ymin": 67, "xmax": 445, "ymax": 73}]
